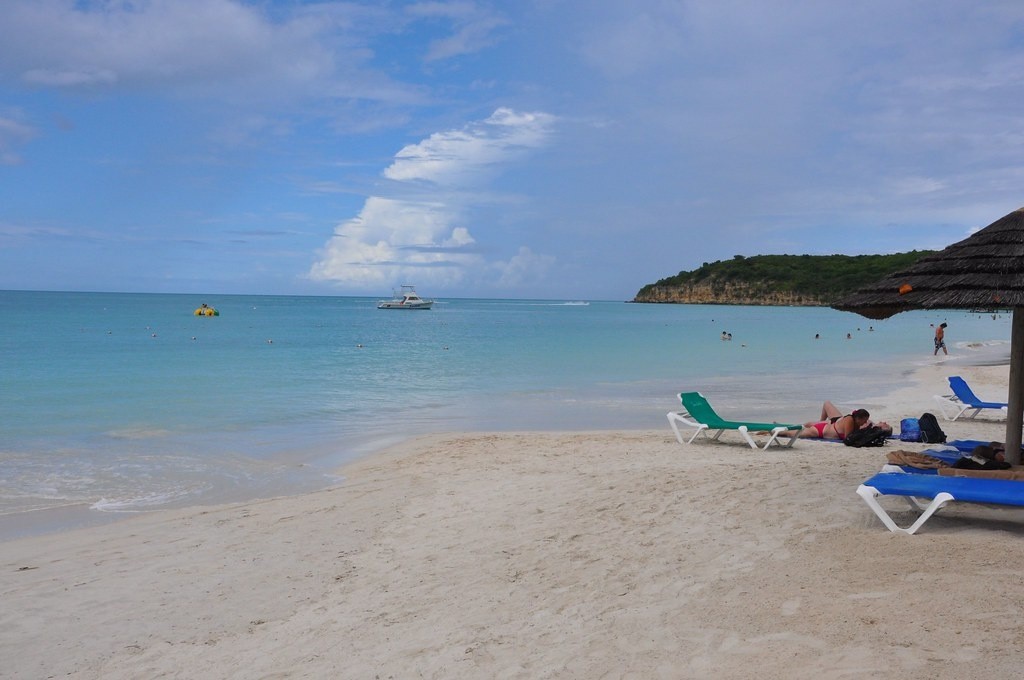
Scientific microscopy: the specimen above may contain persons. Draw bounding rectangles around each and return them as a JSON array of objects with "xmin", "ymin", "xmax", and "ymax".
[
  {"xmin": 815, "ymin": 326, "xmax": 874, "ymax": 339},
  {"xmin": 756, "ymin": 400, "xmax": 893, "ymax": 440},
  {"xmin": 929, "ymin": 322, "xmax": 948, "ymax": 355},
  {"xmin": 972, "ymin": 444, "xmax": 1005, "ymax": 462},
  {"xmin": 721, "ymin": 331, "xmax": 732, "ymax": 342}
]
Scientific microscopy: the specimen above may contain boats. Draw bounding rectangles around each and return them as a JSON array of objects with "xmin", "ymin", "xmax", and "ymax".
[
  {"xmin": 193, "ymin": 303, "xmax": 220, "ymax": 317},
  {"xmin": 376, "ymin": 285, "xmax": 433, "ymax": 309}
]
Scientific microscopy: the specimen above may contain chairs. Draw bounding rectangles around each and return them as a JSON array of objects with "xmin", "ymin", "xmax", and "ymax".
[
  {"xmin": 667, "ymin": 391, "xmax": 804, "ymax": 451},
  {"xmin": 857, "ymin": 440, "xmax": 1024, "ymax": 534},
  {"xmin": 935, "ymin": 376, "xmax": 1009, "ymax": 422}
]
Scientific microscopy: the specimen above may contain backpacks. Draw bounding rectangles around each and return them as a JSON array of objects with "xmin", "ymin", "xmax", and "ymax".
[
  {"xmin": 918, "ymin": 413, "xmax": 947, "ymax": 444},
  {"xmin": 844, "ymin": 423, "xmax": 887, "ymax": 448},
  {"xmin": 900, "ymin": 417, "xmax": 921, "ymax": 441}
]
